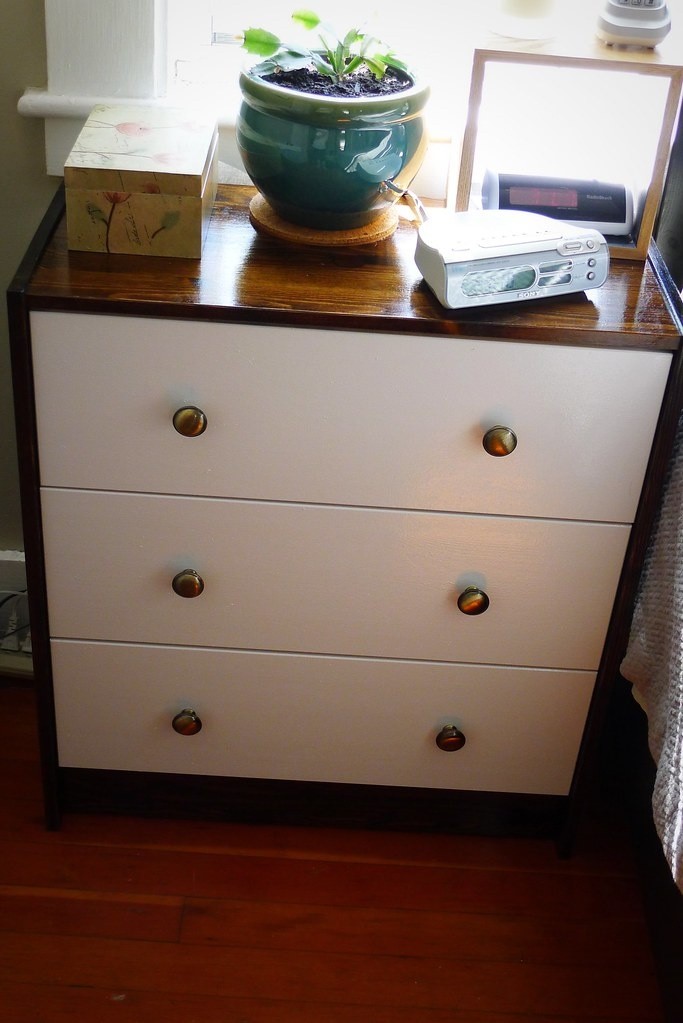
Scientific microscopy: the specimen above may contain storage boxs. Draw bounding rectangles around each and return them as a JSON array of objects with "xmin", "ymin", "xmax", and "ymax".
[{"xmin": 63, "ymin": 103, "xmax": 221, "ymax": 261}]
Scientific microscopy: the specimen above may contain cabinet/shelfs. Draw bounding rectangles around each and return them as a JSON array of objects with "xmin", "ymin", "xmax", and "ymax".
[{"xmin": 3, "ymin": 173, "xmax": 682, "ymax": 844}]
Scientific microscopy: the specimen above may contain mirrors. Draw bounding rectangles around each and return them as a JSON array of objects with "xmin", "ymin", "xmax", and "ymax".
[{"xmin": 454, "ymin": 49, "xmax": 683, "ymax": 261}]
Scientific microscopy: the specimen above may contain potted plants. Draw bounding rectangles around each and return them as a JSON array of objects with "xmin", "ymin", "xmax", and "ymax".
[{"xmin": 237, "ymin": 12, "xmax": 431, "ymax": 246}]
